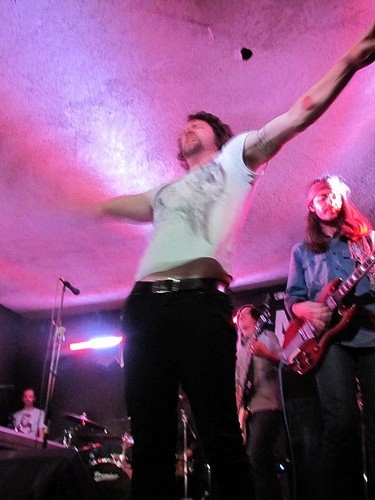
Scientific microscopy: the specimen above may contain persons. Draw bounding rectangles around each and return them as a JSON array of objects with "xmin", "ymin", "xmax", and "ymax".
[
  {"xmin": 285, "ymin": 176, "xmax": 375, "ymax": 500},
  {"xmin": 99, "ymin": 23, "xmax": 375, "ymax": 500},
  {"xmin": 13, "ymin": 388, "xmax": 44, "ymax": 437},
  {"xmin": 236, "ymin": 304, "xmax": 287, "ymax": 500}
]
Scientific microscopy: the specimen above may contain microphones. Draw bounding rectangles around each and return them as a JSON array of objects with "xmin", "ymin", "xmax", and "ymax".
[{"xmin": 61, "ymin": 278, "xmax": 80, "ymax": 295}]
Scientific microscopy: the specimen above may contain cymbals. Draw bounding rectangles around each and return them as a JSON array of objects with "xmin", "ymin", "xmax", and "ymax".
[
  {"xmin": 110, "ymin": 436, "xmax": 134, "ymax": 448},
  {"xmin": 61, "ymin": 411, "xmax": 100, "ymax": 430},
  {"xmin": 76, "ymin": 442, "xmax": 103, "ymax": 451}
]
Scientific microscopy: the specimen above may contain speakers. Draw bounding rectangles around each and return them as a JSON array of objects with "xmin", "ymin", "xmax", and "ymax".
[{"xmin": 0, "ymin": 444, "xmax": 99, "ymax": 500}]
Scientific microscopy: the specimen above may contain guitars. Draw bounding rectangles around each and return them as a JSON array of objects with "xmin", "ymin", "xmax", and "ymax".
[
  {"xmin": 282, "ymin": 250, "xmax": 375, "ymax": 375},
  {"xmin": 236, "ymin": 294, "xmax": 273, "ymax": 451}
]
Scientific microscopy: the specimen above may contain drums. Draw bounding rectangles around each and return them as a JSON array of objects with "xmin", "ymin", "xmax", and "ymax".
[
  {"xmin": 88, "ymin": 459, "xmax": 133, "ymax": 493},
  {"xmin": 175, "ymin": 456, "xmax": 193, "ymax": 476}
]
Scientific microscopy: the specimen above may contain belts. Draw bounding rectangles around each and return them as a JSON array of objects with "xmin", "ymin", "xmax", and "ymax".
[{"xmin": 133, "ymin": 278, "xmax": 229, "ymax": 295}]
